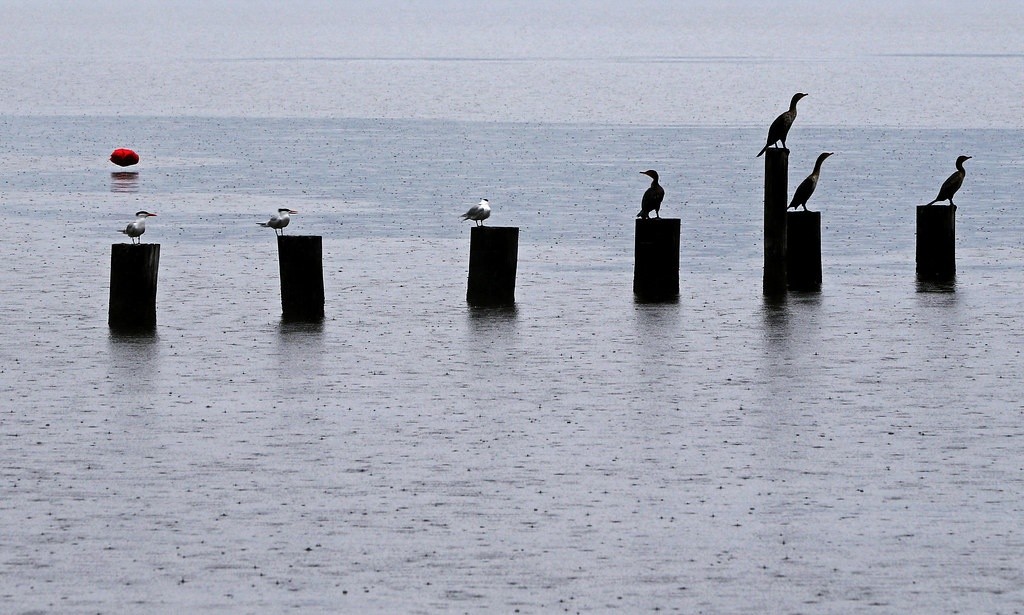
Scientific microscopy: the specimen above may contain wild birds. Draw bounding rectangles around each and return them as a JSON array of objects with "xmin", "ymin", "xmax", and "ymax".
[
  {"xmin": 787, "ymin": 152, "xmax": 834, "ymax": 212},
  {"xmin": 461, "ymin": 198, "xmax": 491, "ymax": 227},
  {"xmin": 756, "ymin": 92, "xmax": 809, "ymax": 158},
  {"xmin": 256, "ymin": 208, "xmax": 299, "ymax": 236},
  {"xmin": 927, "ymin": 155, "xmax": 972, "ymax": 205},
  {"xmin": 636, "ymin": 170, "xmax": 665, "ymax": 219},
  {"xmin": 117, "ymin": 211, "xmax": 157, "ymax": 243}
]
